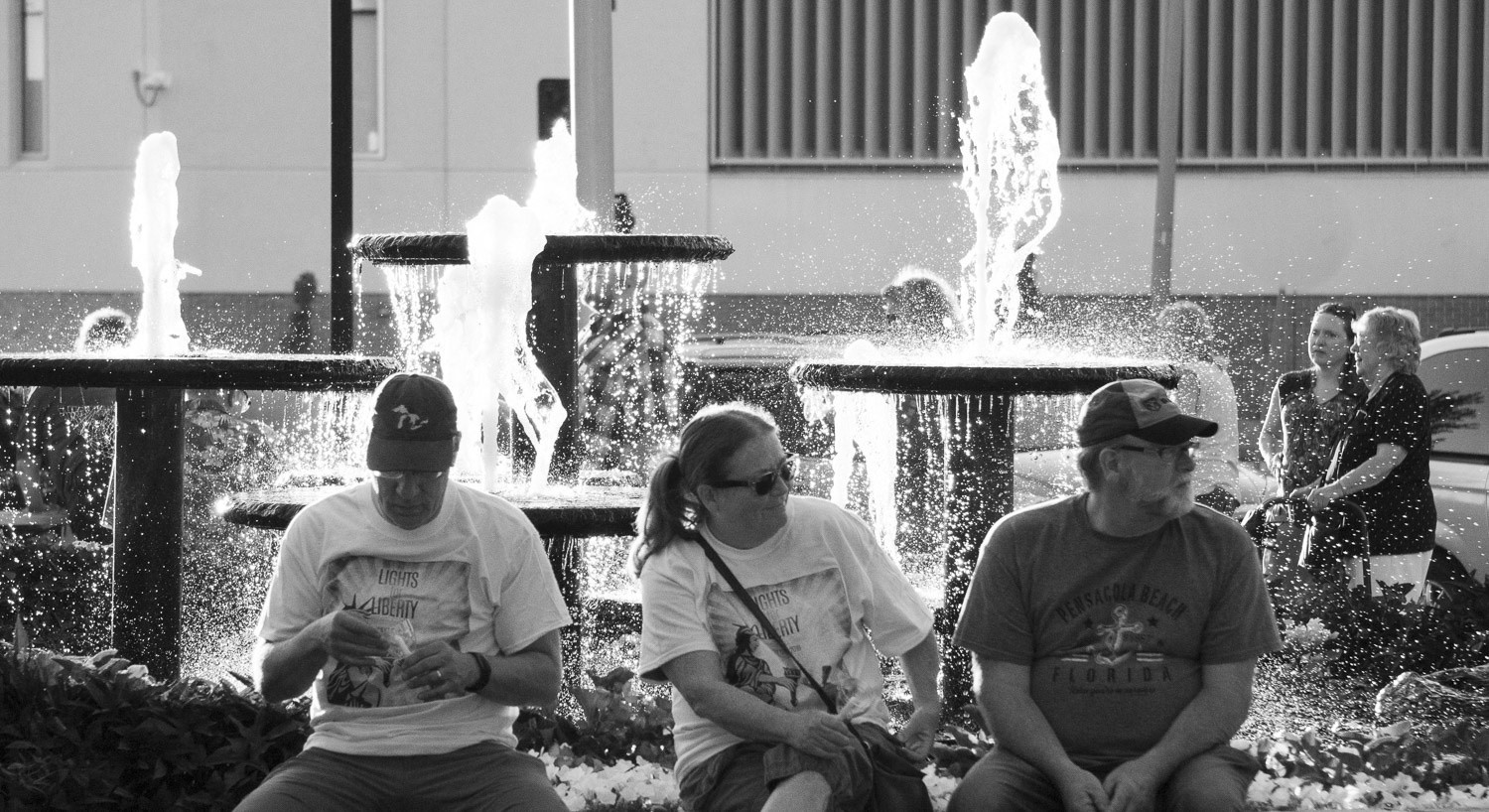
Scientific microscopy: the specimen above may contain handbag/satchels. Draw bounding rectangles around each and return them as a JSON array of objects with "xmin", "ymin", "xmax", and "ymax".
[{"xmin": 868, "ymin": 743, "xmax": 936, "ymax": 812}]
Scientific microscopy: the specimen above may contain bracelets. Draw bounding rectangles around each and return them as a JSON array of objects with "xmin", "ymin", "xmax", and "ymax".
[
  {"xmin": 1339, "ymin": 479, "xmax": 1348, "ymax": 498},
  {"xmin": 464, "ymin": 652, "xmax": 492, "ymax": 693}
]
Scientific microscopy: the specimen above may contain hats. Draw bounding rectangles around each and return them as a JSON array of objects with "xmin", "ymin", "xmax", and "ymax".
[
  {"xmin": 1076, "ymin": 378, "xmax": 1220, "ymax": 448},
  {"xmin": 364, "ymin": 373, "xmax": 458, "ymax": 473}
]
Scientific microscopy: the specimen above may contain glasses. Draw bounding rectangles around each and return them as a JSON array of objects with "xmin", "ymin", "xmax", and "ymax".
[
  {"xmin": 1353, "ymin": 335, "xmax": 1368, "ymax": 348},
  {"xmin": 1108, "ymin": 440, "xmax": 1201, "ymax": 465},
  {"xmin": 371, "ymin": 469, "xmax": 444, "ymax": 480},
  {"xmin": 1319, "ymin": 302, "xmax": 1356, "ymax": 321},
  {"xmin": 704, "ymin": 452, "xmax": 799, "ymax": 498}
]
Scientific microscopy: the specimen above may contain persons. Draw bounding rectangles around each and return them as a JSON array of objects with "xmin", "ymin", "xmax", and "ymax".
[
  {"xmin": 15, "ymin": 309, "xmax": 279, "ymax": 547},
  {"xmin": 632, "ymin": 410, "xmax": 943, "ymax": 812},
  {"xmin": 233, "ymin": 375, "xmax": 573, "ymax": 812},
  {"xmin": 880, "ymin": 275, "xmax": 959, "ymax": 571},
  {"xmin": 1259, "ymin": 303, "xmax": 1438, "ymax": 608},
  {"xmin": 947, "ymin": 378, "xmax": 1284, "ymax": 812},
  {"xmin": 1156, "ymin": 302, "xmax": 1241, "ymax": 518}
]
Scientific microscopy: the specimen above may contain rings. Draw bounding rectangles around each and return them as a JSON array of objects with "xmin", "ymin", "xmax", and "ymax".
[{"xmin": 436, "ymin": 670, "xmax": 443, "ymax": 680}]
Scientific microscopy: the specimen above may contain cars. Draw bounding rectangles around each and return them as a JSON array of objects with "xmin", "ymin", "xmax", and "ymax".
[{"xmin": 1409, "ymin": 324, "xmax": 1489, "ymax": 678}]
